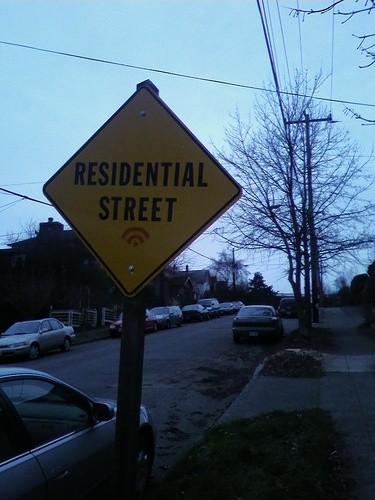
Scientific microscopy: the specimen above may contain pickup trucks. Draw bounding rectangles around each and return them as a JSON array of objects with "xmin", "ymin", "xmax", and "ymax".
[{"xmin": 150, "ymin": 306, "xmax": 184, "ymax": 328}]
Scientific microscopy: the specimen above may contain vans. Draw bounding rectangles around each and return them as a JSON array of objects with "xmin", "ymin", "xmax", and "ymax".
[
  {"xmin": 196, "ymin": 298, "xmax": 221, "ymax": 319},
  {"xmin": 278, "ymin": 297, "xmax": 300, "ymax": 319}
]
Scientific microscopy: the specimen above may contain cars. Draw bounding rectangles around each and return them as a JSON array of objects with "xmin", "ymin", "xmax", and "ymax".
[
  {"xmin": 182, "ymin": 304, "xmax": 209, "ymax": 321},
  {"xmin": 0, "ymin": 317, "xmax": 76, "ymax": 360},
  {"xmin": 220, "ymin": 301, "xmax": 235, "ymax": 316},
  {"xmin": 109, "ymin": 308, "xmax": 157, "ymax": 337},
  {"xmin": 231, "ymin": 300, "xmax": 246, "ymax": 313},
  {"xmin": 0, "ymin": 365, "xmax": 156, "ymax": 499},
  {"xmin": 232, "ymin": 304, "xmax": 284, "ymax": 345}
]
